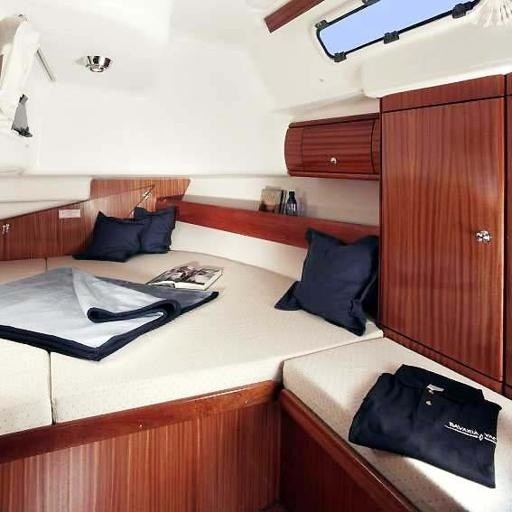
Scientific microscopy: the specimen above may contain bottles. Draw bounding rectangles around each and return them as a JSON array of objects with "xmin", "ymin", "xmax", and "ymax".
[{"xmin": 285, "ymin": 191, "xmax": 297, "ymax": 216}]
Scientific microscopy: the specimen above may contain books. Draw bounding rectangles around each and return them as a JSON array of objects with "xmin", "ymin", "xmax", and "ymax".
[{"xmin": 146, "ymin": 263, "xmax": 224, "ymax": 291}]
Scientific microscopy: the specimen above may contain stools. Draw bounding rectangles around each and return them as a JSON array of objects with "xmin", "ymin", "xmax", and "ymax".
[{"xmin": 277, "ymin": 339, "xmax": 510, "ymax": 512}]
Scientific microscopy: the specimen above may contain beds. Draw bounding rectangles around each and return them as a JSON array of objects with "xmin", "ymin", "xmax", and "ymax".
[{"xmin": 0, "ymin": 176, "xmax": 387, "ymax": 512}]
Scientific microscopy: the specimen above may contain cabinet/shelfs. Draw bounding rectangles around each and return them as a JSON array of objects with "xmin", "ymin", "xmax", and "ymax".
[
  {"xmin": 282, "ymin": 114, "xmax": 381, "ymax": 176},
  {"xmin": 377, "ymin": 97, "xmax": 508, "ymax": 396}
]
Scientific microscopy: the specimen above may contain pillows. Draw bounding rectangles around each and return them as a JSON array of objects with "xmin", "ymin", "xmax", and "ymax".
[
  {"xmin": 273, "ymin": 226, "xmax": 379, "ymax": 337},
  {"xmin": 71, "ymin": 205, "xmax": 179, "ymax": 263}
]
